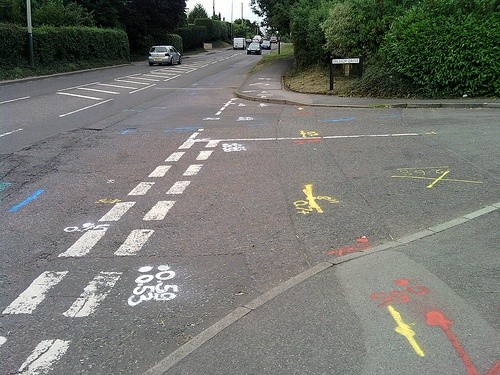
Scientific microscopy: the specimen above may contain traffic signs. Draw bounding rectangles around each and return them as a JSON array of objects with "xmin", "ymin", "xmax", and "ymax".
[{"xmin": 332, "ymin": 58, "xmax": 360, "ymax": 64}]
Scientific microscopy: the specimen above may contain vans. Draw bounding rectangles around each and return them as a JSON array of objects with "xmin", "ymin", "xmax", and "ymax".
[{"xmin": 233, "ymin": 38, "xmax": 247, "ymax": 50}]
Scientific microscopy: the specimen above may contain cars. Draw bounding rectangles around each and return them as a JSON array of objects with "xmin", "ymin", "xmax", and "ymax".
[
  {"xmin": 245, "ymin": 39, "xmax": 251, "ymax": 43},
  {"xmin": 247, "ymin": 43, "xmax": 261, "ymax": 54},
  {"xmin": 270, "ymin": 36, "xmax": 278, "ymax": 44},
  {"xmin": 252, "ymin": 35, "xmax": 262, "ymax": 43},
  {"xmin": 261, "ymin": 41, "xmax": 272, "ymax": 50},
  {"xmin": 148, "ymin": 46, "xmax": 182, "ymax": 66}
]
